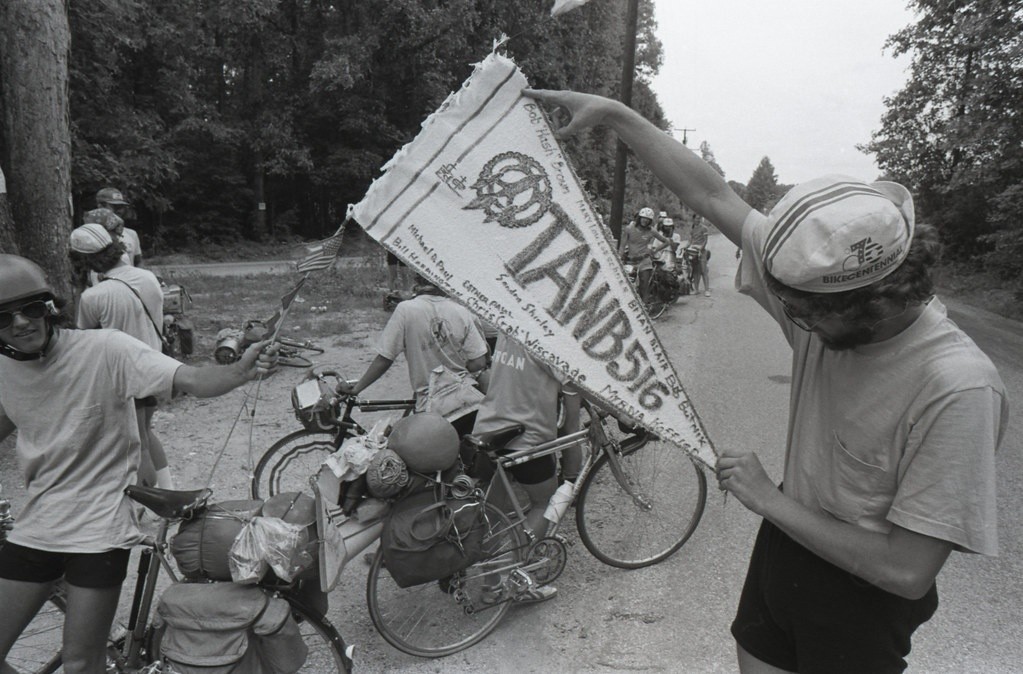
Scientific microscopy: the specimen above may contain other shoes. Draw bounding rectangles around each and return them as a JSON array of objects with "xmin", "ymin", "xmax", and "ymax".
[
  {"xmin": 705, "ymin": 289, "xmax": 711, "ymax": 297},
  {"xmin": 690, "ymin": 290, "xmax": 699, "ymax": 295}
]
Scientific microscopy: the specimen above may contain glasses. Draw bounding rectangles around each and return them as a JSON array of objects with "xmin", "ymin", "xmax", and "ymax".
[
  {"xmin": 0, "ymin": 300, "xmax": 51, "ymax": 330},
  {"xmin": 765, "ymin": 280, "xmax": 857, "ymax": 333}
]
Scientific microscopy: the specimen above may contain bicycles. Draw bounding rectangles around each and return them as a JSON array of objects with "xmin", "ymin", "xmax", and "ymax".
[
  {"xmin": 367, "ymin": 399, "xmax": 708, "ymax": 658},
  {"xmin": 241, "ymin": 319, "xmax": 325, "ymax": 369},
  {"xmin": 0, "ymin": 486, "xmax": 358, "ymax": 674},
  {"xmin": 627, "ymin": 260, "xmax": 680, "ymax": 319},
  {"xmin": 252, "ymin": 370, "xmax": 417, "ymax": 528},
  {"xmin": 679, "ymin": 247, "xmax": 711, "ymax": 296}
]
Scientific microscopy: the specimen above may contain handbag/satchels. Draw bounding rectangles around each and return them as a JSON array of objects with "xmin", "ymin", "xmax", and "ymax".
[
  {"xmin": 384, "ymin": 490, "xmax": 503, "ymax": 587},
  {"xmin": 154, "ymin": 577, "xmax": 307, "ymax": 674}
]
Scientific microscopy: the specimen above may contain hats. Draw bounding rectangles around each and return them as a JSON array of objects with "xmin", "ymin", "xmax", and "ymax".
[
  {"xmin": 757, "ymin": 175, "xmax": 919, "ymax": 295},
  {"xmin": 70, "ymin": 226, "xmax": 113, "ymax": 254},
  {"xmin": 660, "ymin": 211, "xmax": 668, "ymax": 217},
  {"xmin": 96, "ymin": 188, "xmax": 131, "ymax": 207},
  {"xmin": 663, "ymin": 217, "xmax": 673, "ymax": 226}
]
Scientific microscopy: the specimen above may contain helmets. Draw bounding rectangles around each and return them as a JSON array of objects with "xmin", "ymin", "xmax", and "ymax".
[
  {"xmin": 0, "ymin": 253, "xmax": 52, "ymax": 304},
  {"xmin": 640, "ymin": 208, "xmax": 654, "ymax": 219}
]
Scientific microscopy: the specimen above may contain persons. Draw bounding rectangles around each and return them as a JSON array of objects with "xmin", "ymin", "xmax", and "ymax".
[
  {"xmin": 521, "ymin": 86, "xmax": 1011, "ymax": 674},
  {"xmin": 680, "ymin": 212, "xmax": 711, "ymax": 296},
  {"xmin": 384, "ymin": 250, "xmax": 410, "ymax": 292},
  {"xmin": 69, "ymin": 188, "xmax": 174, "ymax": 525},
  {"xmin": 473, "ymin": 332, "xmax": 583, "ymax": 604},
  {"xmin": 0, "ymin": 253, "xmax": 279, "ymax": 674},
  {"xmin": 334, "ymin": 269, "xmax": 498, "ymax": 492},
  {"xmin": 620, "ymin": 207, "xmax": 682, "ymax": 306}
]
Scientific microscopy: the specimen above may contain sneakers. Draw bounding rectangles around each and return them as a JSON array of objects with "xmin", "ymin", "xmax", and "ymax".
[
  {"xmin": 483, "ymin": 572, "xmax": 510, "ymax": 604},
  {"xmin": 502, "ymin": 569, "xmax": 558, "ymax": 604}
]
